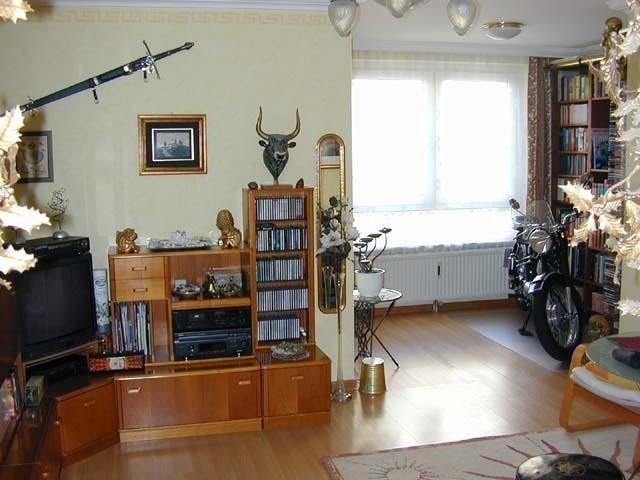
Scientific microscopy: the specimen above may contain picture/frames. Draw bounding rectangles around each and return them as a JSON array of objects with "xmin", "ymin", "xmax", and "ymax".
[
  {"xmin": 7, "ymin": 130, "xmax": 55, "ymax": 183},
  {"xmin": 136, "ymin": 113, "xmax": 208, "ymax": 176}
]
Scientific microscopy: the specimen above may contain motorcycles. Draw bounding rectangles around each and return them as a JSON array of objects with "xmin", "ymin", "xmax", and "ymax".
[{"xmin": 503, "ymin": 197, "xmax": 585, "ymax": 362}]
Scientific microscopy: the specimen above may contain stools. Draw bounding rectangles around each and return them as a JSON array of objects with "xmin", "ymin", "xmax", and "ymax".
[{"xmin": 357, "ymin": 357, "xmax": 387, "ymax": 396}]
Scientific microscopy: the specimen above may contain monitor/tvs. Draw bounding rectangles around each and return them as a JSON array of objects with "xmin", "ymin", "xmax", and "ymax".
[{"xmin": 14, "ymin": 252, "xmax": 98, "ymax": 363}]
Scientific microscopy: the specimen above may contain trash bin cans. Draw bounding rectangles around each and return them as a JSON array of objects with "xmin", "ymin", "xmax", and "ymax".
[{"xmin": 359, "ymin": 356, "xmax": 390, "ymax": 397}]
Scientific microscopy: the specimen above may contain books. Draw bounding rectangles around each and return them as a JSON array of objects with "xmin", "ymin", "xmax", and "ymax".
[
  {"xmin": 591, "ymin": 127, "xmax": 612, "ymax": 170},
  {"xmin": 109, "ymin": 301, "xmax": 150, "ymax": 354},
  {"xmin": 566, "ymin": 238, "xmax": 582, "ymax": 279},
  {"xmin": 560, "ymin": 103, "xmax": 588, "ymax": 126},
  {"xmin": 560, "ymin": 127, "xmax": 588, "ymax": 151},
  {"xmin": 601, "ymin": 287, "xmax": 619, "ymax": 319},
  {"xmin": 560, "ymin": 154, "xmax": 586, "ymax": 176},
  {"xmin": 593, "ymin": 64, "xmax": 610, "ymax": 99},
  {"xmin": 588, "ymin": 228, "xmax": 612, "ymax": 251},
  {"xmin": 554, "ymin": 208, "xmax": 584, "ymax": 237},
  {"xmin": 557, "ymin": 178, "xmax": 612, "ymax": 204},
  {"xmin": 553, "ymin": 69, "xmax": 589, "ymax": 99},
  {"xmin": 592, "ymin": 254, "xmax": 618, "ymax": 287}
]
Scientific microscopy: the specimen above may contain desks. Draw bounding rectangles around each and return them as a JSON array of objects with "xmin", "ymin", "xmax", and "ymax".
[
  {"xmin": 557, "ymin": 332, "xmax": 640, "ymax": 472},
  {"xmin": 353, "ymin": 287, "xmax": 403, "ymax": 373}
]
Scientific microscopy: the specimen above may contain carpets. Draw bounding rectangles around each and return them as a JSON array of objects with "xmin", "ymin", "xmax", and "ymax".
[{"xmin": 321, "ymin": 419, "xmax": 639, "ymax": 479}]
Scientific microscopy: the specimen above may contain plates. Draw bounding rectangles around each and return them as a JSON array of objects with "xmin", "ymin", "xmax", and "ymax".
[
  {"xmin": 149, "ymin": 236, "xmax": 219, "ymax": 251},
  {"xmin": 173, "ymin": 283, "xmax": 201, "ymax": 299}
]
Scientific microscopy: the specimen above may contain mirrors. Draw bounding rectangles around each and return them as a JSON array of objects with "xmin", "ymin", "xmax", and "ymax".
[{"xmin": 314, "ymin": 131, "xmax": 347, "ymax": 314}]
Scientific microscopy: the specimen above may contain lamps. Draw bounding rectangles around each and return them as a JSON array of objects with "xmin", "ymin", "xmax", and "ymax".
[
  {"xmin": 446, "ymin": 0, "xmax": 478, "ymax": 37},
  {"xmin": 326, "ymin": 0, "xmax": 359, "ymax": 38},
  {"xmin": 374, "ymin": 0, "xmax": 422, "ymax": 21},
  {"xmin": 481, "ymin": 20, "xmax": 526, "ymax": 42}
]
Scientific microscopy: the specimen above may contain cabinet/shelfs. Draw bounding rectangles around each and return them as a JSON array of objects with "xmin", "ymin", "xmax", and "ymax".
[
  {"xmin": 0, "ymin": 385, "xmax": 60, "ymax": 480},
  {"xmin": 58, "ymin": 376, "xmax": 119, "ymax": 463},
  {"xmin": 543, "ymin": 54, "xmax": 640, "ymax": 335},
  {"xmin": 106, "ymin": 187, "xmax": 337, "ymax": 443}
]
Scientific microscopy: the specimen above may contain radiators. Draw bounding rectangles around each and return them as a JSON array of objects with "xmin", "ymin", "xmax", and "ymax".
[{"xmin": 354, "ymin": 247, "xmax": 514, "ymax": 312}]
